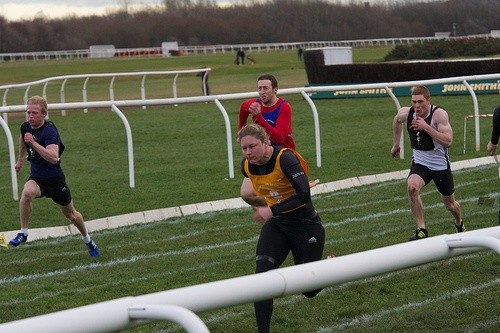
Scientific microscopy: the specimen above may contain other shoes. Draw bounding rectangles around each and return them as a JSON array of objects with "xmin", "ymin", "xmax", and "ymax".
[{"xmin": 327, "ymin": 253, "xmax": 336, "ymax": 259}]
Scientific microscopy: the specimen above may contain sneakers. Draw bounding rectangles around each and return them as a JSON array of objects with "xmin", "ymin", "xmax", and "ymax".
[
  {"xmin": 9, "ymin": 233, "xmax": 28, "ymax": 247},
  {"xmin": 408, "ymin": 228, "xmax": 428, "ymax": 241},
  {"xmin": 455, "ymin": 218, "xmax": 466, "ymax": 233},
  {"xmin": 85, "ymin": 240, "xmax": 99, "ymax": 257}
]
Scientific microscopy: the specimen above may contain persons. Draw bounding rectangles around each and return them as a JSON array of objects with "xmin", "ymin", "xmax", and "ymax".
[
  {"xmin": 239, "ymin": 124, "xmax": 336, "ymax": 333},
  {"xmin": 297, "ymin": 46, "xmax": 303, "ymax": 62},
  {"xmin": 488, "ymin": 105, "xmax": 500, "ymax": 157},
  {"xmin": 237, "ymin": 47, "xmax": 245, "ymax": 65},
  {"xmin": 390, "ymin": 86, "xmax": 467, "ymax": 242},
  {"xmin": 236, "ymin": 75, "xmax": 320, "ymax": 221},
  {"xmin": 9, "ymin": 96, "xmax": 100, "ymax": 257}
]
